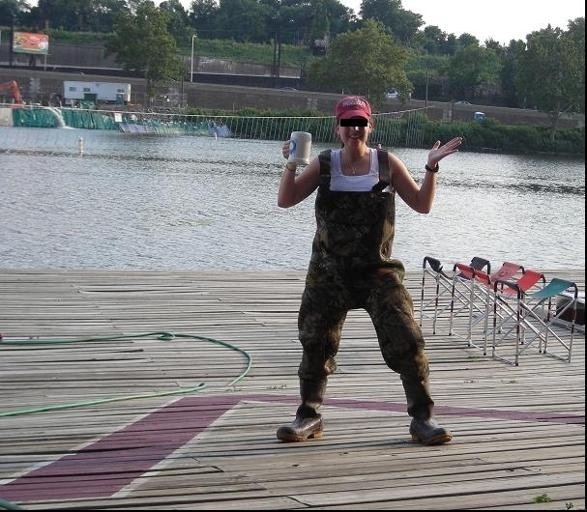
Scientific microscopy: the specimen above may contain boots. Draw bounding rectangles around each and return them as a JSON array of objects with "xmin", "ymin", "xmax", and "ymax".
[
  {"xmin": 277, "ymin": 380, "xmax": 325, "ymax": 442},
  {"xmin": 409, "ymin": 402, "xmax": 451, "ymax": 445}
]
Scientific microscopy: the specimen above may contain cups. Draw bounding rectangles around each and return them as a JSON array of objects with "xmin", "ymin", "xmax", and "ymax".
[{"xmin": 286, "ymin": 131, "xmax": 312, "ymax": 165}]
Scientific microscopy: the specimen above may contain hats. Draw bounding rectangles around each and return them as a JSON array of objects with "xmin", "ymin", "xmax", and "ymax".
[{"xmin": 336, "ymin": 97, "xmax": 370, "ymax": 124}]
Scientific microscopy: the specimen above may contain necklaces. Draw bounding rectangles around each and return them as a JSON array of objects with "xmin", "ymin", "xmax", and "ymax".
[{"xmin": 350, "ymin": 166, "xmax": 359, "ymax": 177}]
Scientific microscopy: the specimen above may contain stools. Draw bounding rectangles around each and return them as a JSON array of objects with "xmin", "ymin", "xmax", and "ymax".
[{"xmin": 423, "ymin": 255, "xmax": 579, "ymax": 366}]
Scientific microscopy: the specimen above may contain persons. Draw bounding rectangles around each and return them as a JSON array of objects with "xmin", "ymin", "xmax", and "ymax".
[{"xmin": 276, "ymin": 93, "xmax": 464, "ymax": 445}]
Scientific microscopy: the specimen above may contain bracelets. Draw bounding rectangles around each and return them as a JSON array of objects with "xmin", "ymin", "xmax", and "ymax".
[
  {"xmin": 285, "ymin": 164, "xmax": 296, "ymax": 174},
  {"xmin": 425, "ymin": 163, "xmax": 441, "ymax": 173}
]
